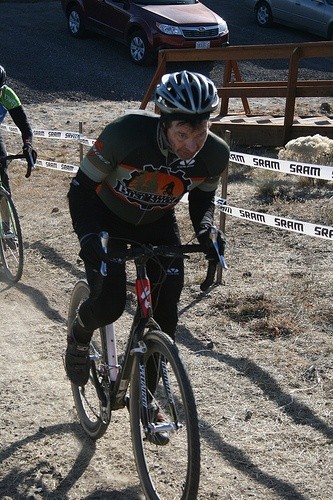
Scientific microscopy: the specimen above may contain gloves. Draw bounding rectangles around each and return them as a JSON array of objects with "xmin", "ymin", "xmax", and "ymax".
[
  {"xmin": 22, "ymin": 143, "xmax": 37, "ymax": 167},
  {"xmin": 198, "ymin": 230, "xmax": 226, "ymax": 261},
  {"xmin": 79, "ymin": 237, "xmax": 108, "ymax": 271}
]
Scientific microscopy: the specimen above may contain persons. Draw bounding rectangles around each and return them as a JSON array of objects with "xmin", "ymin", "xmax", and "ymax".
[
  {"xmin": 66, "ymin": 71, "xmax": 230, "ymax": 444},
  {"xmin": 0, "ymin": 66, "xmax": 34, "ymax": 240}
]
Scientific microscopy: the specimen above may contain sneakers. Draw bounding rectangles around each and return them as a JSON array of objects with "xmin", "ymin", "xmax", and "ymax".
[
  {"xmin": 141, "ymin": 404, "xmax": 169, "ymax": 445},
  {"xmin": 65, "ymin": 318, "xmax": 91, "ymax": 386}
]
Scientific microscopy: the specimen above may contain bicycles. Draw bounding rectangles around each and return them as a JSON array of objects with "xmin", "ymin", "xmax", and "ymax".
[
  {"xmin": 0, "ymin": 145, "xmax": 38, "ymax": 282},
  {"xmin": 68, "ymin": 224, "xmax": 230, "ymax": 500}
]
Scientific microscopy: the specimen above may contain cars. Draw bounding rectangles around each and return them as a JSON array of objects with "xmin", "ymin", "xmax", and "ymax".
[
  {"xmin": 244, "ymin": 0, "xmax": 333, "ymax": 42},
  {"xmin": 65, "ymin": 0, "xmax": 230, "ymax": 66}
]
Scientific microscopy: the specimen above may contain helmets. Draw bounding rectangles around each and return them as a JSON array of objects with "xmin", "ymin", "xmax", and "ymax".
[
  {"xmin": 0, "ymin": 65, "xmax": 7, "ymax": 91},
  {"xmin": 155, "ymin": 70, "xmax": 219, "ymax": 113}
]
